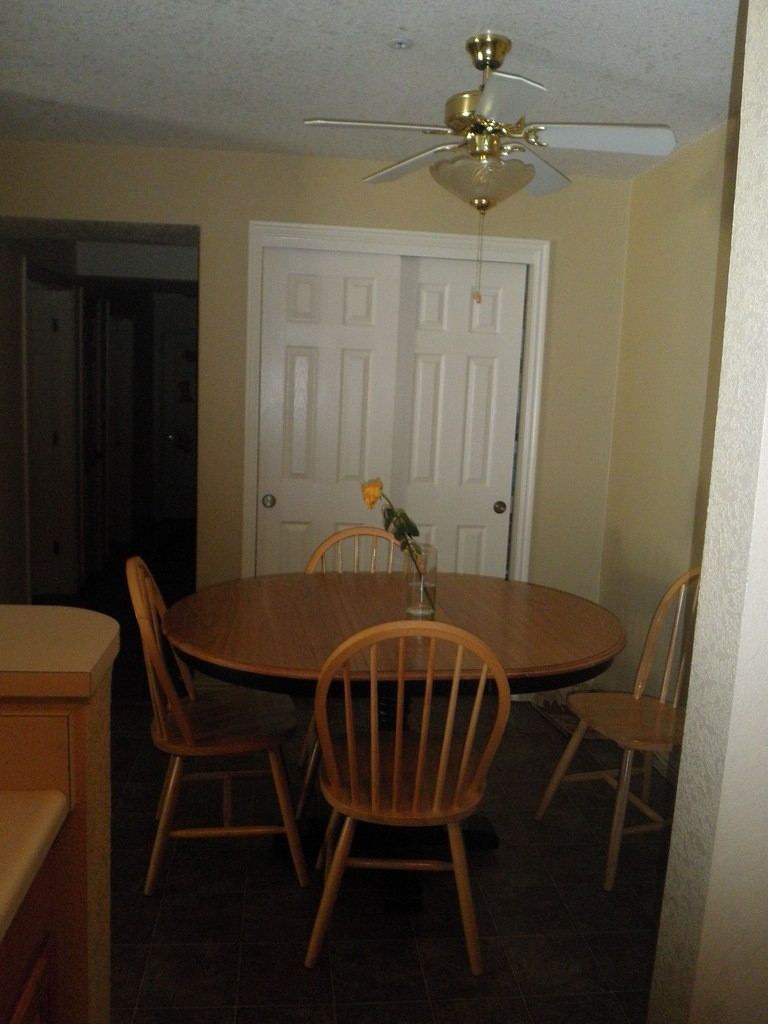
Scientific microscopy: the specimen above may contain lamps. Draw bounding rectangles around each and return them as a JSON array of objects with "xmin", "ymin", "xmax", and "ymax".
[{"xmin": 430, "ymin": 156, "xmax": 535, "ymax": 211}]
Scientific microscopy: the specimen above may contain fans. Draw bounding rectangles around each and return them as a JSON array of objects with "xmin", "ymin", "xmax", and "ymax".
[{"xmin": 304, "ymin": 32, "xmax": 677, "ymax": 195}]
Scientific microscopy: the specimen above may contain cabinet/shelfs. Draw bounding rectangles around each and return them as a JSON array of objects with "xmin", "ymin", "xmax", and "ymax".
[{"xmin": 0, "ymin": 603, "xmax": 121, "ymax": 1024}]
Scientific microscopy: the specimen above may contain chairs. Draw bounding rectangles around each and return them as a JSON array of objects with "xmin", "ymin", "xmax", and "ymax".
[
  {"xmin": 303, "ymin": 620, "xmax": 510, "ymax": 977},
  {"xmin": 125, "ymin": 557, "xmax": 311, "ymax": 895},
  {"xmin": 290, "ymin": 525, "xmax": 425, "ymax": 823},
  {"xmin": 534, "ymin": 565, "xmax": 701, "ymax": 892}
]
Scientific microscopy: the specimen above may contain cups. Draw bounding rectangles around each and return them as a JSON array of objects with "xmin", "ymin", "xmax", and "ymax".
[{"xmin": 404, "ymin": 545, "xmax": 438, "ymax": 618}]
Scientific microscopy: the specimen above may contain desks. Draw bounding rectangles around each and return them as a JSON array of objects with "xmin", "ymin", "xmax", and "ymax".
[{"xmin": 160, "ymin": 572, "xmax": 629, "ymax": 914}]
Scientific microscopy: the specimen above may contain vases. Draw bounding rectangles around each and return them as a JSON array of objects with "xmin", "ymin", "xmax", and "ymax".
[{"xmin": 403, "ymin": 542, "xmax": 439, "ymax": 619}]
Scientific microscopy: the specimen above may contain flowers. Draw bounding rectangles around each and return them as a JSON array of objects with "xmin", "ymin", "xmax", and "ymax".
[{"xmin": 361, "ymin": 481, "xmax": 435, "ymax": 613}]
